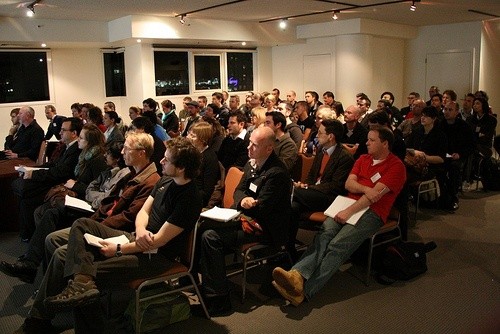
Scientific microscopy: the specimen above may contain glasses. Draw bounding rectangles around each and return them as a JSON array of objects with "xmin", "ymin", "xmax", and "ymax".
[{"xmin": 60, "ymin": 128, "xmax": 71, "ymax": 131}]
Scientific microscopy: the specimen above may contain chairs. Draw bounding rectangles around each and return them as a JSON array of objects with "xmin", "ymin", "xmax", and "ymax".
[{"xmin": 29, "ymin": 108, "xmax": 496, "ymax": 334}]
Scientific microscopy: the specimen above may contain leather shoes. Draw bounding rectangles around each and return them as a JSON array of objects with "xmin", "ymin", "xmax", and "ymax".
[
  {"xmin": 0, "ymin": 258, "xmax": 38, "ymax": 284},
  {"xmin": 449, "ymin": 202, "xmax": 459, "ymax": 210}
]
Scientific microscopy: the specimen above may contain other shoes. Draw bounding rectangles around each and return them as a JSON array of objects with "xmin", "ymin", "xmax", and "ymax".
[
  {"xmin": 195, "ymin": 296, "xmax": 232, "ymax": 316},
  {"xmin": 272, "ymin": 267, "xmax": 305, "ymax": 307}
]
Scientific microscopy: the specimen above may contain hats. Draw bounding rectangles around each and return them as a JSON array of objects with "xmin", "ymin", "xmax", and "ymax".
[{"xmin": 185, "ymin": 101, "xmax": 199, "ymax": 106}]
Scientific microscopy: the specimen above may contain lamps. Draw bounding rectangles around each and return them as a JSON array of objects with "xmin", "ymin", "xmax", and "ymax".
[
  {"xmin": 27, "ymin": 5, "xmax": 34, "ymax": 17},
  {"xmin": 410, "ymin": 0, "xmax": 416, "ymax": 12},
  {"xmin": 332, "ymin": 10, "xmax": 338, "ymax": 20},
  {"xmin": 178, "ymin": 14, "xmax": 187, "ymax": 25}
]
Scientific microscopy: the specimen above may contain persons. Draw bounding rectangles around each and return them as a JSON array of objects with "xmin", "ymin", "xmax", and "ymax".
[
  {"xmin": 215, "ymin": 111, "xmax": 251, "ymax": 168},
  {"xmin": 337, "ymin": 105, "xmax": 366, "ymax": 158},
  {"xmin": 395, "ymin": 106, "xmax": 446, "ymax": 217},
  {"xmin": 272, "ymin": 127, "xmax": 406, "ymax": 305},
  {"xmin": 0, "ymin": 86, "xmax": 497, "ymax": 334},
  {"xmin": 161, "ymin": 100, "xmax": 179, "ymax": 133},
  {"xmin": 43, "ymin": 104, "xmax": 68, "ymax": 141},
  {"xmin": 185, "ymin": 126, "xmax": 291, "ymax": 296}
]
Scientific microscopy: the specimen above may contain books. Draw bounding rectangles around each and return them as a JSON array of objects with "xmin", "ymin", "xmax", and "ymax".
[
  {"xmin": 200, "ymin": 206, "xmax": 241, "ymax": 222},
  {"xmin": 14, "ymin": 164, "xmax": 49, "ymax": 172},
  {"xmin": 323, "ymin": 195, "xmax": 369, "ymax": 225},
  {"xmin": 64, "ymin": 194, "xmax": 95, "ymax": 212},
  {"xmin": 84, "ymin": 233, "xmax": 130, "ymax": 248}
]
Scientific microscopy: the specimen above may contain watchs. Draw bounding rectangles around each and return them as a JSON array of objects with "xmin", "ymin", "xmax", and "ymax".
[{"xmin": 116, "ymin": 243, "xmax": 122, "ymax": 256}]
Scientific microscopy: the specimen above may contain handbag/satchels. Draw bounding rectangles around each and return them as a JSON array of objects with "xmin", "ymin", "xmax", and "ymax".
[
  {"xmin": 123, "ymin": 285, "xmax": 191, "ymax": 333},
  {"xmin": 461, "ymin": 175, "xmax": 483, "ymax": 193},
  {"xmin": 44, "ymin": 183, "xmax": 77, "ymax": 208},
  {"xmin": 375, "ymin": 241, "xmax": 437, "ymax": 287},
  {"xmin": 405, "ymin": 148, "xmax": 427, "ymax": 171}
]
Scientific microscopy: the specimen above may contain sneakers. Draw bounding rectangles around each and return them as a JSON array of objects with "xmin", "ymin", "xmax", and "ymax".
[
  {"xmin": 48, "ymin": 279, "xmax": 101, "ymax": 309},
  {"xmin": 12, "ymin": 317, "xmax": 54, "ymax": 334}
]
